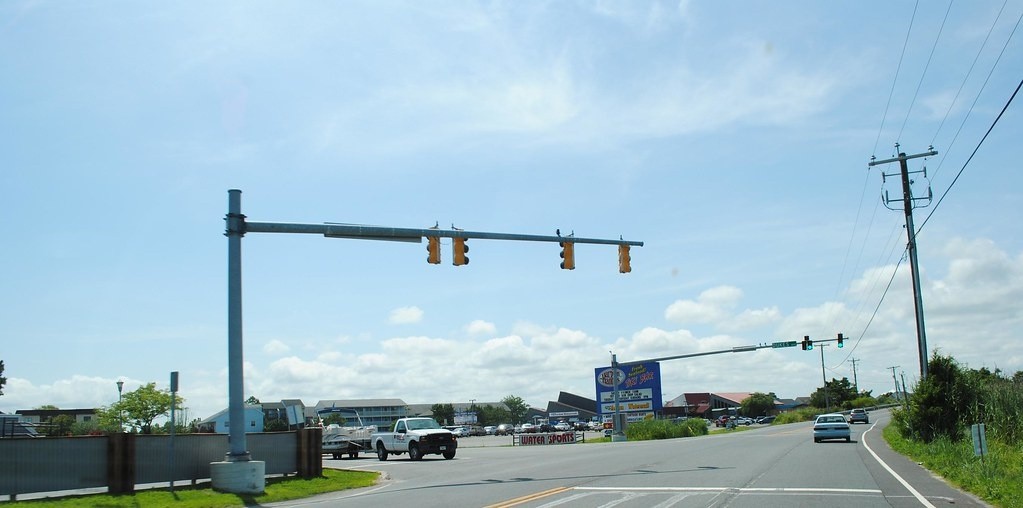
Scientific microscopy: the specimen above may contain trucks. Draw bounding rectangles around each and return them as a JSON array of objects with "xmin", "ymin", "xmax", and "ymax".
[{"xmin": 307, "ymin": 406, "xmax": 379, "ymax": 461}]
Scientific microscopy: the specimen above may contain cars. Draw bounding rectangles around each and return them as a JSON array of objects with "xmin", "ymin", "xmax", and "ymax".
[
  {"xmin": 849, "ymin": 408, "xmax": 869, "ymax": 424},
  {"xmin": 573, "ymin": 421, "xmax": 602, "ymax": 432},
  {"xmin": 671, "ymin": 417, "xmax": 712, "ymax": 426},
  {"xmin": 452, "ymin": 428, "xmax": 471, "ymax": 438},
  {"xmin": 514, "ymin": 422, "xmax": 557, "ymax": 434},
  {"xmin": 757, "ymin": 416, "xmax": 775, "ymax": 425},
  {"xmin": 484, "ymin": 426, "xmax": 497, "ymax": 435},
  {"xmin": 715, "ymin": 415, "xmax": 766, "ymax": 428},
  {"xmin": 469, "ymin": 426, "xmax": 486, "ymax": 436},
  {"xmin": 554, "ymin": 422, "xmax": 571, "ymax": 432},
  {"xmin": 813, "ymin": 413, "xmax": 850, "ymax": 443}
]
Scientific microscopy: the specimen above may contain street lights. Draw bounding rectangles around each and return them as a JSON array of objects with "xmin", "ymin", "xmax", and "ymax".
[{"xmin": 116, "ymin": 379, "xmax": 124, "ymax": 433}]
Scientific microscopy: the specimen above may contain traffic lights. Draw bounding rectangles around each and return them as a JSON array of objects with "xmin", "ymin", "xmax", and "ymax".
[
  {"xmin": 452, "ymin": 228, "xmax": 469, "ymax": 265},
  {"xmin": 560, "ymin": 235, "xmax": 576, "ymax": 270},
  {"xmin": 838, "ymin": 334, "xmax": 843, "ymax": 347},
  {"xmin": 808, "ymin": 341, "xmax": 812, "ymax": 350}
]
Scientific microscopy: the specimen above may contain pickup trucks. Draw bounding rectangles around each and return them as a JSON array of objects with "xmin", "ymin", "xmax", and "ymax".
[{"xmin": 371, "ymin": 417, "xmax": 457, "ymax": 461}]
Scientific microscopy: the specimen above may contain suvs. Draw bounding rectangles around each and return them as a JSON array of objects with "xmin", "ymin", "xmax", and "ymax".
[{"xmin": 495, "ymin": 424, "xmax": 515, "ymax": 437}]
